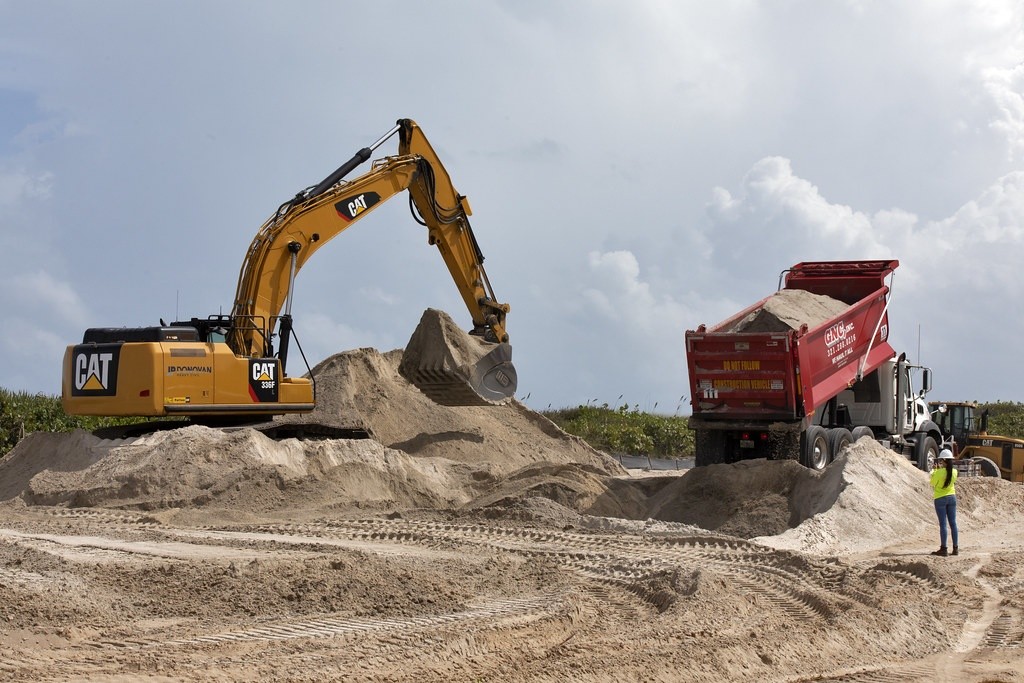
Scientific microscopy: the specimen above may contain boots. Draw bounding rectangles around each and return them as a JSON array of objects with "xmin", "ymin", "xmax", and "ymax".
[
  {"xmin": 950, "ymin": 545, "xmax": 959, "ymax": 555},
  {"xmin": 932, "ymin": 546, "xmax": 948, "ymax": 556}
]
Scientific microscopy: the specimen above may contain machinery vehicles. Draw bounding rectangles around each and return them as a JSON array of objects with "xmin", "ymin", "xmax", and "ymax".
[
  {"xmin": 61, "ymin": 116, "xmax": 521, "ymax": 425},
  {"xmin": 928, "ymin": 400, "xmax": 1024, "ymax": 484}
]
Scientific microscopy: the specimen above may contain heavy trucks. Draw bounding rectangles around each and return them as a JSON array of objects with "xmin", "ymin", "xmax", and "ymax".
[{"xmin": 683, "ymin": 261, "xmax": 943, "ymax": 472}]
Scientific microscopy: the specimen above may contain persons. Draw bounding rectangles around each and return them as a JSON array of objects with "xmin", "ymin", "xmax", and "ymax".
[{"xmin": 929, "ymin": 449, "xmax": 958, "ymax": 556}]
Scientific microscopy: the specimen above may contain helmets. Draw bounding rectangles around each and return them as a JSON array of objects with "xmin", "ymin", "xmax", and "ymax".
[{"xmin": 938, "ymin": 449, "xmax": 955, "ymax": 460}]
